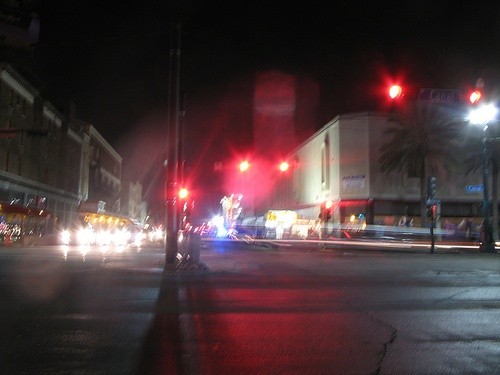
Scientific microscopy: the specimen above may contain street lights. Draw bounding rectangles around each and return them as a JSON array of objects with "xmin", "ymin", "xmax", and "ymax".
[{"xmin": 475, "ymin": 106, "xmax": 496, "ymax": 254}]
